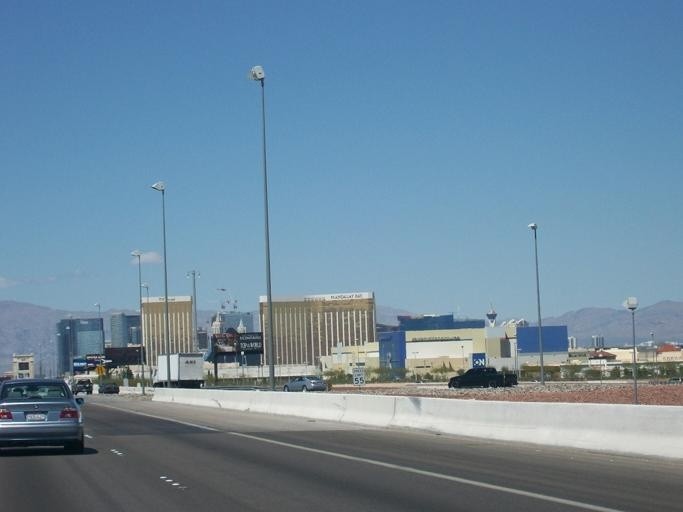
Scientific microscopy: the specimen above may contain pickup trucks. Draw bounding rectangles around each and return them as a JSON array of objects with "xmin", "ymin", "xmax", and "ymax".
[{"xmin": 447, "ymin": 366, "xmax": 518, "ymax": 388}]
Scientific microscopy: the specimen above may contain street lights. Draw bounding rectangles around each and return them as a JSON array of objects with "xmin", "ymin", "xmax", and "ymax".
[
  {"xmin": 151, "ymin": 181, "xmax": 172, "ymax": 388},
  {"xmin": 93, "ymin": 248, "xmax": 145, "ymax": 387},
  {"xmin": 248, "ymin": 63, "xmax": 276, "ymax": 393},
  {"xmin": 527, "ymin": 222, "xmax": 545, "ymax": 385},
  {"xmin": 627, "ymin": 295, "xmax": 639, "ymax": 405}
]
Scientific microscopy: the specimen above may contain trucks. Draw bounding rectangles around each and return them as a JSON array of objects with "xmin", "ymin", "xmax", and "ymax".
[{"xmin": 152, "ymin": 351, "xmax": 208, "ymax": 388}]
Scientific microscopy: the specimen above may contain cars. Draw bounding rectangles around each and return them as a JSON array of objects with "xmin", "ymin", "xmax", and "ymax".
[
  {"xmin": 72, "ymin": 378, "xmax": 119, "ymax": 396},
  {"xmin": 282, "ymin": 376, "xmax": 326, "ymax": 393},
  {"xmin": 0, "ymin": 377, "xmax": 85, "ymax": 454}
]
